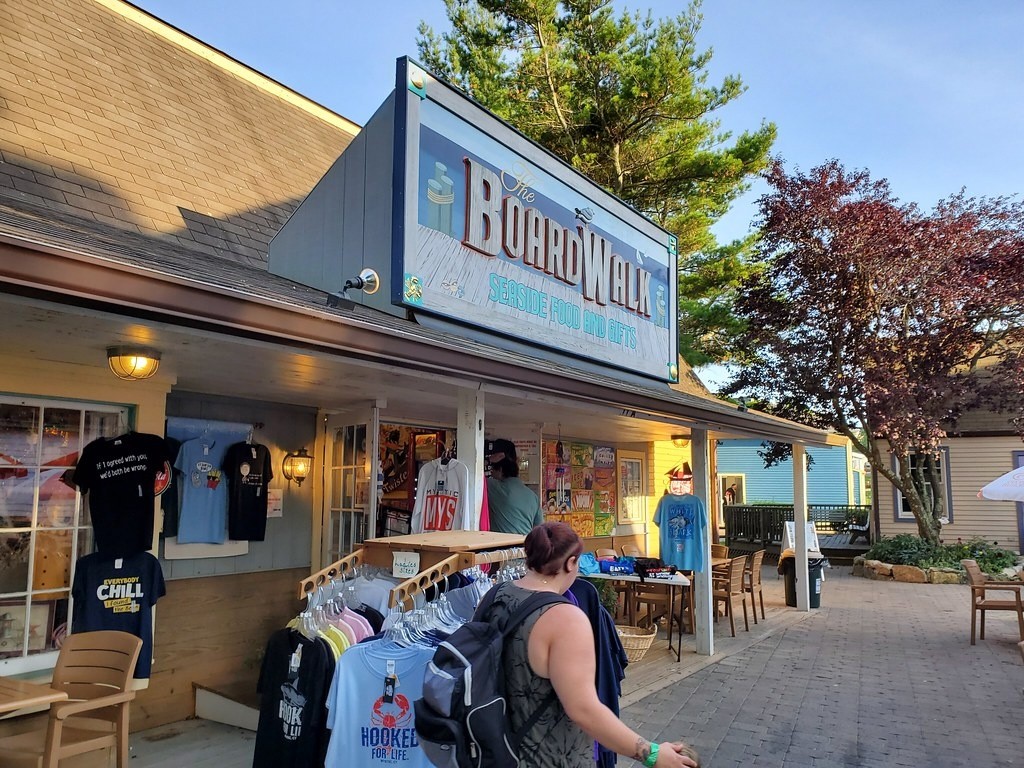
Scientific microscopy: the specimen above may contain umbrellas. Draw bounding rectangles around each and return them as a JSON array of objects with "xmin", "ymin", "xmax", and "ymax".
[
  {"xmin": 0, "ymin": 454, "xmax": 83, "ymax": 502},
  {"xmin": 977, "ymin": 466, "xmax": 1024, "ymax": 502}
]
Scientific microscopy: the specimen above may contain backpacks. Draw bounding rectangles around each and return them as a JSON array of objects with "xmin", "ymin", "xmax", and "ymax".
[{"xmin": 414, "ymin": 582, "xmax": 574, "ymax": 768}]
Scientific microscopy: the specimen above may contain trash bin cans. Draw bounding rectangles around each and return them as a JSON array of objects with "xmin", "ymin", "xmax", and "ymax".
[{"xmin": 779, "ymin": 548, "xmax": 828, "ymax": 607}]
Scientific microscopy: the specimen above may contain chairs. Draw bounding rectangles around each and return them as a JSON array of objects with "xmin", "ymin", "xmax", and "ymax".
[
  {"xmin": 595, "ymin": 542, "xmax": 766, "ymax": 639},
  {"xmin": 0, "ymin": 629, "xmax": 144, "ymax": 768},
  {"xmin": 961, "ymin": 559, "xmax": 1024, "ymax": 645}
]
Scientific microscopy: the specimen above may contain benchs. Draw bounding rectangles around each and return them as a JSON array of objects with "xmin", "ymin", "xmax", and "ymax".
[{"xmin": 848, "ymin": 511, "xmax": 871, "ymax": 544}]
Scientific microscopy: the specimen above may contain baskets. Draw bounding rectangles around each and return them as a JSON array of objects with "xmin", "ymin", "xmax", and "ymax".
[{"xmin": 614, "ymin": 623, "xmax": 657, "ymax": 662}]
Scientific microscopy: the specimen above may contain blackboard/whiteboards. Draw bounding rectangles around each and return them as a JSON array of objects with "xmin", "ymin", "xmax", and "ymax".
[{"xmin": 780, "ymin": 522, "xmax": 821, "ymax": 551}]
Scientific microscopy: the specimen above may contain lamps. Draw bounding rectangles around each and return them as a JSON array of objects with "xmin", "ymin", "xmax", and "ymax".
[
  {"xmin": 671, "ymin": 435, "xmax": 691, "ymax": 448},
  {"xmin": 282, "ymin": 446, "xmax": 312, "ymax": 486},
  {"xmin": 105, "ymin": 345, "xmax": 162, "ymax": 381},
  {"xmin": 328, "ymin": 269, "xmax": 381, "ymax": 307}
]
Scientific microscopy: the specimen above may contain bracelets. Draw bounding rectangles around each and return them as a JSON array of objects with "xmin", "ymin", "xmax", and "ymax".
[{"xmin": 642, "ymin": 743, "xmax": 659, "ymax": 768}]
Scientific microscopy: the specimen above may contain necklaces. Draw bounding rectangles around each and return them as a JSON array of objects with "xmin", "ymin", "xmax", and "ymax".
[{"xmin": 525, "ymin": 575, "xmax": 558, "ymax": 590}]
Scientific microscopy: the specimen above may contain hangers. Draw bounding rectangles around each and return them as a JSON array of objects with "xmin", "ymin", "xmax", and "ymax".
[
  {"xmin": 288, "ymin": 563, "xmax": 390, "ymax": 642},
  {"xmin": 245, "ymin": 427, "xmax": 257, "ymax": 446},
  {"xmin": 436, "ymin": 447, "xmax": 452, "ymax": 465},
  {"xmin": 376, "ymin": 574, "xmax": 472, "ymax": 650},
  {"xmin": 471, "ymin": 547, "xmax": 532, "ymax": 593},
  {"xmin": 671, "ymin": 487, "xmax": 687, "ymax": 496},
  {"xmin": 104, "ymin": 411, "xmax": 131, "ymax": 440},
  {"xmin": 196, "ymin": 422, "xmax": 216, "ymax": 447}
]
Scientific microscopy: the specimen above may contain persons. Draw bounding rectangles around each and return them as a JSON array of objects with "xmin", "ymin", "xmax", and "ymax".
[
  {"xmin": 723, "ymin": 484, "xmax": 737, "ymax": 505},
  {"xmin": 484, "ymin": 452, "xmax": 543, "ymax": 579},
  {"xmin": 472, "ymin": 522, "xmax": 698, "ymax": 768},
  {"xmin": 670, "ymin": 742, "xmax": 702, "ymax": 768}
]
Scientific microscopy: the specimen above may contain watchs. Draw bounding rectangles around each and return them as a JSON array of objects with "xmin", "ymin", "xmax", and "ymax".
[{"xmin": 485, "ymin": 454, "xmax": 492, "ymax": 465}]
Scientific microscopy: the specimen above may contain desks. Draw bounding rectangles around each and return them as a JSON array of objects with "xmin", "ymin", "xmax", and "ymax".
[
  {"xmin": 713, "ymin": 556, "xmax": 732, "ymax": 622},
  {"xmin": 0, "ymin": 675, "xmax": 68, "ymax": 718},
  {"xmin": 576, "ymin": 563, "xmax": 691, "ymax": 662}
]
